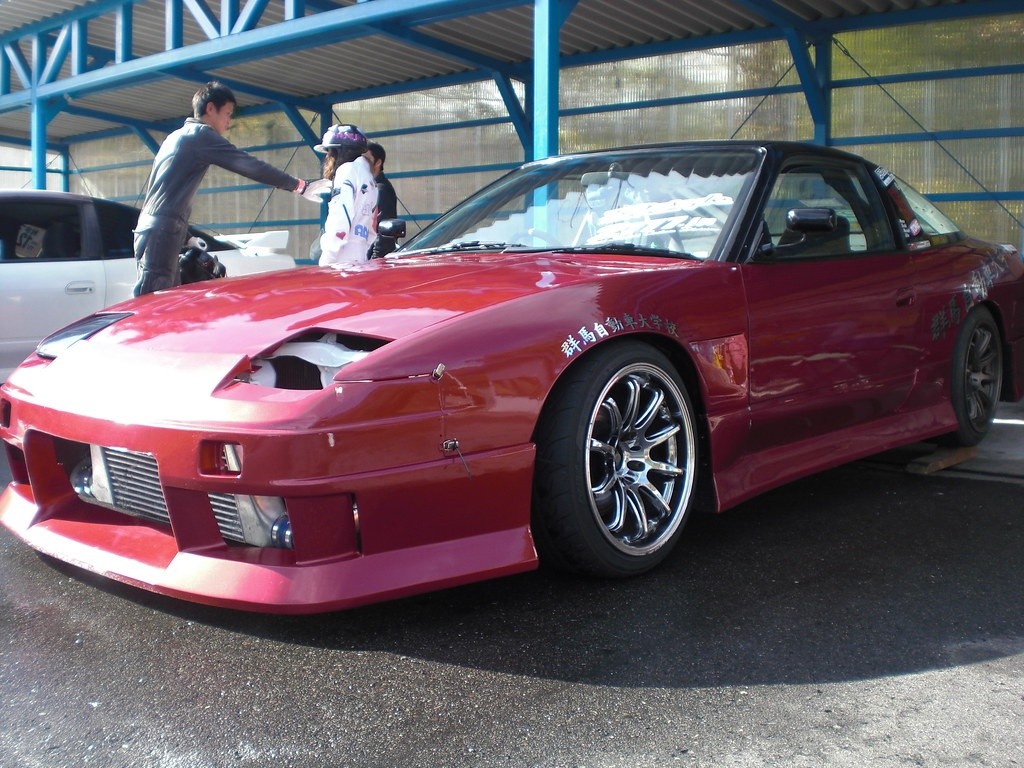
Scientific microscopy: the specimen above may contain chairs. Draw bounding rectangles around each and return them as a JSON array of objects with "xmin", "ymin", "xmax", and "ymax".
[{"xmin": 776, "ymin": 216, "xmax": 850, "ymax": 255}]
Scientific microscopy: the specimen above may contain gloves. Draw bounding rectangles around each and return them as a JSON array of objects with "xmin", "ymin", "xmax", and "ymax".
[
  {"xmin": 300, "ymin": 179, "xmax": 332, "ymax": 203},
  {"xmin": 188, "ymin": 236, "xmax": 207, "ymax": 251}
]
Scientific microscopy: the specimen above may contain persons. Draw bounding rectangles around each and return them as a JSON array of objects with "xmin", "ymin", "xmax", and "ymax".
[
  {"xmin": 133, "ymin": 80, "xmax": 332, "ymax": 298},
  {"xmin": 363, "ymin": 141, "xmax": 397, "ymax": 260},
  {"xmin": 314, "ymin": 124, "xmax": 377, "ymax": 266}
]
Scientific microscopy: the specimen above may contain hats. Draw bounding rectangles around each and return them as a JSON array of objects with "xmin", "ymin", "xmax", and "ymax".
[{"xmin": 314, "ymin": 124, "xmax": 367, "ymax": 153}]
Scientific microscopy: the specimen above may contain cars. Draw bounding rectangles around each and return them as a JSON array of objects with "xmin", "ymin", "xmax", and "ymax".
[{"xmin": 0, "ymin": 189, "xmax": 296, "ymax": 385}]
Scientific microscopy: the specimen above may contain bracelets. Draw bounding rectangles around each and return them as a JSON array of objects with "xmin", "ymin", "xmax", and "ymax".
[{"xmin": 301, "ymin": 180, "xmax": 309, "ymax": 195}]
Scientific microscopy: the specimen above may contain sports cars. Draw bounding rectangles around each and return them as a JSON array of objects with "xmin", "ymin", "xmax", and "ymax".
[{"xmin": 1, "ymin": 141, "xmax": 1024, "ymax": 614}]
